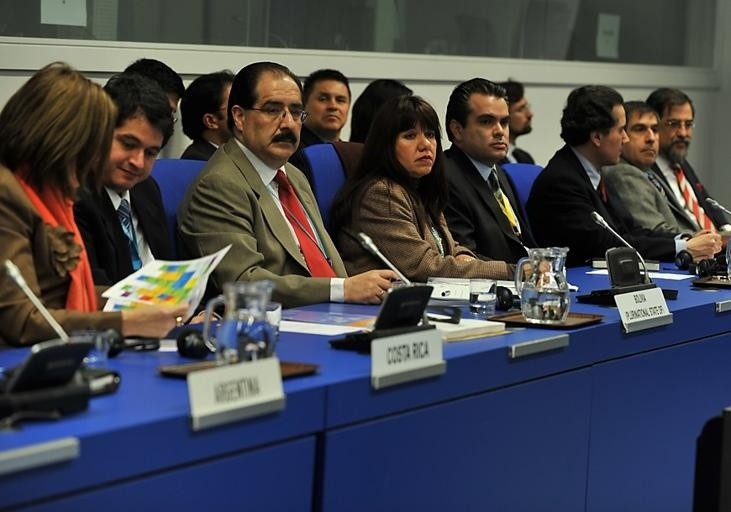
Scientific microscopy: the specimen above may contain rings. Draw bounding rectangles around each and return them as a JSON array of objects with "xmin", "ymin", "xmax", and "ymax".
[
  {"xmin": 375, "ymin": 289, "xmax": 386, "ymax": 296},
  {"xmin": 174, "ymin": 315, "xmax": 184, "ymax": 328}
]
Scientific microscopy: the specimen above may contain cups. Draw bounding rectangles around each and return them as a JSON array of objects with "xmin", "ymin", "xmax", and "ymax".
[
  {"xmin": 202, "ymin": 278, "xmax": 277, "ymax": 364},
  {"xmin": 517, "ymin": 247, "xmax": 572, "ymax": 325},
  {"xmin": 73, "ymin": 330, "xmax": 108, "ymax": 369},
  {"xmin": 265, "ymin": 303, "xmax": 282, "ymax": 334},
  {"xmin": 468, "ymin": 277, "xmax": 498, "ymax": 318}
]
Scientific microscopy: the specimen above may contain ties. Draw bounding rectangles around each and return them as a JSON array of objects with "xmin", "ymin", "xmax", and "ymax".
[
  {"xmin": 672, "ymin": 161, "xmax": 718, "ymax": 233},
  {"xmin": 118, "ymin": 198, "xmax": 143, "ymax": 271},
  {"xmin": 646, "ymin": 173, "xmax": 665, "ymax": 198},
  {"xmin": 597, "ymin": 174, "xmax": 609, "ymax": 203},
  {"xmin": 273, "ymin": 170, "xmax": 337, "ymax": 278},
  {"xmin": 488, "ymin": 169, "xmax": 522, "ymax": 237}
]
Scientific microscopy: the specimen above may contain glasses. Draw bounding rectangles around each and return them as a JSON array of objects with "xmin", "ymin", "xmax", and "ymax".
[
  {"xmin": 244, "ymin": 106, "xmax": 309, "ymax": 122},
  {"xmin": 663, "ymin": 118, "xmax": 695, "ymax": 129}
]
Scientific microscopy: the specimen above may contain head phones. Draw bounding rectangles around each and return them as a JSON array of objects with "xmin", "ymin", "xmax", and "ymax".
[
  {"xmin": 177, "ymin": 327, "xmax": 215, "ymax": 359},
  {"xmin": 489, "ymin": 284, "xmax": 520, "ymax": 310},
  {"xmin": 675, "ymin": 251, "xmax": 693, "ymax": 270},
  {"xmin": 99, "ymin": 329, "xmax": 160, "ymax": 358},
  {"xmin": 696, "ymin": 258, "xmax": 727, "ymax": 277}
]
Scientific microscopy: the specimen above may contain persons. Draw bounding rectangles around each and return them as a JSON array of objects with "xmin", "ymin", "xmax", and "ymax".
[
  {"xmin": 72, "ymin": 74, "xmax": 227, "ymax": 326},
  {"xmin": 327, "ymin": 95, "xmax": 552, "ymax": 285},
  {"xmin": 179, "ymin": 69, "xmax": 236, "ymax": 162},
  {"xmin": 288, "ymin": 68, "xmax": 350, "ymax": 177},
  {"xmin": 497, "ymin": 82, "xmax": 536, "ymax": 164},
  {"xmin": 647, "ymin": 88, "xmax": 731, "ymax": 236},
  {"xmin": 351, "ymin": 77, "xmax": 412, "ymax": 141},
  {"xmin": 1, "ymin": 59, "xmax": 186, "ymax": 346},
  {"xmin": 525, "ymin": 85, "xmax": 724, "ymax": 270},
  {"xmin": 177, "ymin": 63, "xmax": 400, "ymax": 309},
  {"xmin": 435, "ymin": 79, "xmax": 541, "ymax": 263},
  {"xmin": 122, "ymin": 60, "xmax": 185, "ymax": 123},
  {"xmin": 601, "ymin": 100, "xmax": 704, "ymax": 239}
]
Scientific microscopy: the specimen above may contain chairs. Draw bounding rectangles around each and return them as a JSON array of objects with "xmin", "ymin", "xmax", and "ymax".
[
  {"xmin": 148, "ymin": 156, "xmax": 216, "ymax": 263},
  {"xmin": 299, "ymin": 139, "xmax": 352, "ymax": 224},
  {"xmin": 500, "ymin": 161, "xmax": 549, "ymax": 243}
]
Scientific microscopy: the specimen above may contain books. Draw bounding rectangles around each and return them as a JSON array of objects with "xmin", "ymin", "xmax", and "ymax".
[{"xmin": 338, "ymin": 313, "xmax": 508, "ymax": 341}]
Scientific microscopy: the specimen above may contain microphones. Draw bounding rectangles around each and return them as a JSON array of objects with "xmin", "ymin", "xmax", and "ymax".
[
  {"xmin": 576, "ymin": 211, "xmax": 656, "ymax": 306},
  {"xmin": 1, "ymin": 258, "xmax": 94, "ymax": 430},
  {"xmin": 705, "ymin": 197, "xmax": 731, "ymax": 215},
  {"xmin": 328, "ymin": 232, "xmax": 436, "ymax": 355}
]
